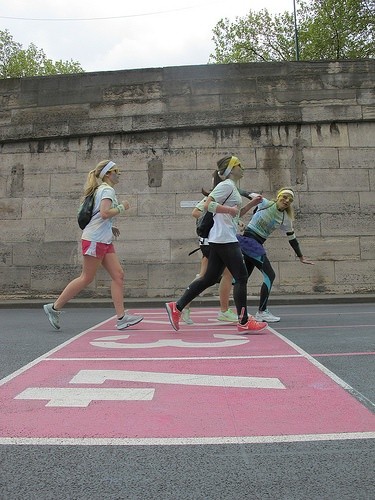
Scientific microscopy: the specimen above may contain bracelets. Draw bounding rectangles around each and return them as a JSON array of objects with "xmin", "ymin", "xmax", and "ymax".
[{"xmin": 116, "ymin": 205, "xmax": 126, "ymax": 214}]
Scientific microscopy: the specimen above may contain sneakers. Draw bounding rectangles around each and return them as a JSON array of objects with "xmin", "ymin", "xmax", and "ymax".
[
  {"xmin": 256, "ymin": 311, "xmax": 281, "ymax": 323},
  {"xmin": 181, "ymin": 307, "xmax": 193, "ymax": 323},
  {"xmin": 43, "ymin": 303, "xmax": 60, "ymax": 328},
  {"xmin": 117, "ymin": 313, "xmax": 144, "ymax": 329},
  {"xmin": 218, "ymin": 309, "xmax": 238, "ymax": 322},
  {"xmin": 165, "ymin": 301, "xmax": 179, "ymax": 332},
  {"xmin": 236, "ymin": 320, "xmax": 268, "ymax": 334}
]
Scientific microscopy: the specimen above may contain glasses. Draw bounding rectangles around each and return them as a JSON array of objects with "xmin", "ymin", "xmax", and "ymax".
[
  {"xmin": 233, "ymin": 163, "xmax": 242, "ymax": 168},
  {"xmin": 107, "ymin": 168, "xmax": 119, "ymax": 174},
  {"xmin": 280, "ymin": 194, "xmax": 294, "ymax": 202}
]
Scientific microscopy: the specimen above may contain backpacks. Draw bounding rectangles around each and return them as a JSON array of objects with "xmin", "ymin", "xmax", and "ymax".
[
  {"xmin": 196, "ymin": 190, "xmax": 233, "ymax": 238},
  {"xmin": 77, "ymin": 184, "xmax": 108, "ymax": 229}
]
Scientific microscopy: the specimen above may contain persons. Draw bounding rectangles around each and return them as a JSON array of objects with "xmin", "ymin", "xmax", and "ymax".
[
  {"xmin": 179, "ymin": 169, "xmax": 241, "ymax": 325},
  {"xmin": 164, "ymin": 156, "xmax": 269, "ymax": 336},
  {"xmin": 42, "ymin": 159, "xmax": 145, "ymax": 332},
  {"xmin": 233, "ymin": 185, "xmax": 315, "ymax": 324}
]
